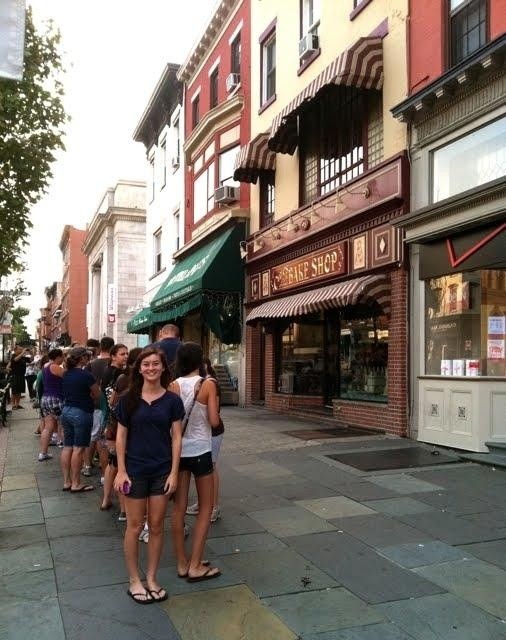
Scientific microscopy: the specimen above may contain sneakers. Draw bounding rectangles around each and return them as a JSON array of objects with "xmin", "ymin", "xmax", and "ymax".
[
  {"xmin": 34, "ymin": 429, "xmax": 64, "ymax": 448},
  {"xmin": 185, "ymin": 500, "xmax": 201, "ymax": 516},
  {"xmin": 11, "ymin": 404, "xmax": 25, "ymax": 410},
  {"xmin": 117, "ymin": 511, "xmax": 127, "ymax": 521},
  {"xmin": 183, "ymin": 523, "xmax": 192, "ymax": 539},
  {"xmin": 210, "ymin": 504, "xmax": 222, "ymax": 523},
  {"xmin": 80, "ymin": 462, "xmax": 105, "ymax": 488},
  {"xmin": 137, "ymin": 522, "xmax": 150, "ymax": 544},
  {"xmin": 37, "ymin": 452, "xmax": 53, "ymax": 462}
]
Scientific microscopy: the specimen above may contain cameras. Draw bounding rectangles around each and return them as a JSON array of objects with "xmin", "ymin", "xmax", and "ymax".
[{"xmin": 120, "ymin": 482, "xmax": 130, "ymax": 495}]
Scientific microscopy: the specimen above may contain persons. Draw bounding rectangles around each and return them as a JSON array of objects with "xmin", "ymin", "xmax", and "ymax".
[{"xmin": 0, "ymin": 323, "xmax": 225, "ymax": 605}]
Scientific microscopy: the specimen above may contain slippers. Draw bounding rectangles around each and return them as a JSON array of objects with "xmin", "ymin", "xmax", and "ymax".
[
  {"xmin": 126, "ymin": 584, "xmax": 169, "ymax": 605},
  {"xmin": 177, "ymin": 561, "xmax": 223, "ymax": 583},
  {"xmin": 61, "ymin": 480, "xmax": 94, "ymax": 493},
  {"xmin": 99, "ymin": 500, "xmax": 113, "ymax": 511}
]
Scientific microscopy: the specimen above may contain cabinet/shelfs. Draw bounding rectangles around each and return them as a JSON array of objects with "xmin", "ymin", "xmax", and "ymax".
[{"xmin": 429, "ymin": 273, "xmax": 482, "ymax": 376}]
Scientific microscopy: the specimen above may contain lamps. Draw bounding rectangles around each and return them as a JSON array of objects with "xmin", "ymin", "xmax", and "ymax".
[
  {"xmin": 335, "ymin": 186, "xmax": 370, "ymax": 215},
  {"xmin": 254, "ymin": 233, "xmax": 273, "ymax": 253},
  {"xmin": 240, "ymin": 241, "xmax": 253, "ymax": 260},
  {"xmin": 287, "ymin": 210, "xmax": 311, "ymax": 232},
  {"xmin": 271, "ymin": 227, "xmax": 287, "ymax": 240},
  {"xmin": 311, "ymin": 200, "xmax": 335, "ymax": 225}
]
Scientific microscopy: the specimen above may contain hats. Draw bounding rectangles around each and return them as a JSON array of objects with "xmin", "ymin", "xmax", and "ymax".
[{"xmin": 69, "ymin": 346, "xmax": 93, "ymax": 359}]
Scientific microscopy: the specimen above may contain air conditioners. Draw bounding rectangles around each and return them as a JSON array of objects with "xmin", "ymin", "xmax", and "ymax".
[
  {"xmin": 171, "ymin": 156, "xmax": 180, "ymax": 168},
  {"xmin": 298, "ymin": 34, "xmax": 319, "ymax": 60},
  {"xmin": 214, "ymin": 185, "xmax": 240, "ymax": 204},
  {"xmin": 226, "ymin": 73, "xmax": 240, "ymax": 92}
]
